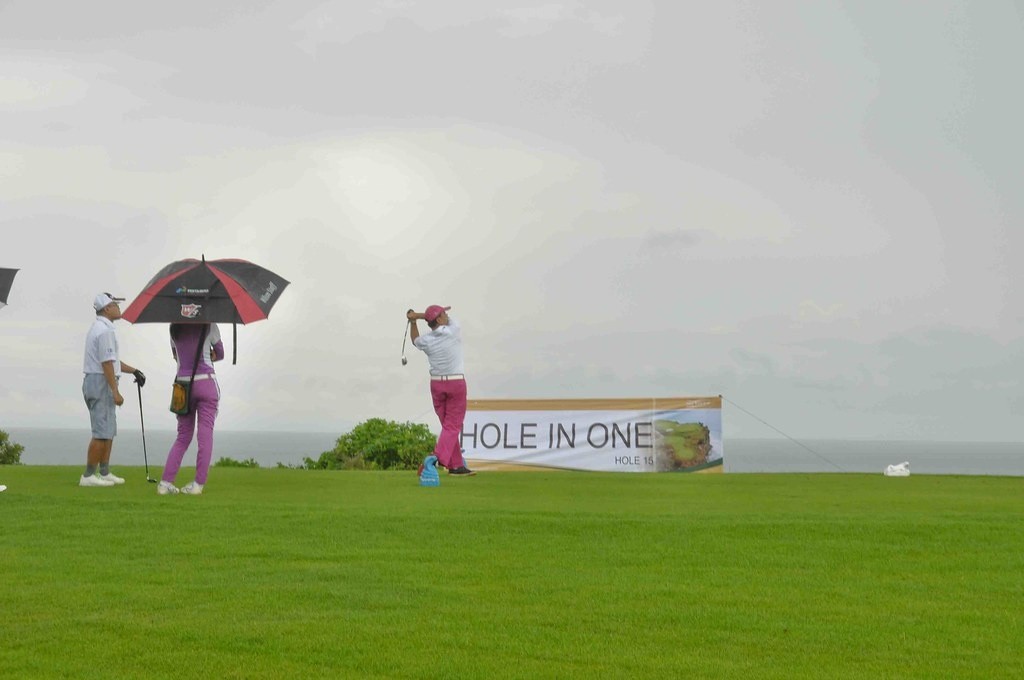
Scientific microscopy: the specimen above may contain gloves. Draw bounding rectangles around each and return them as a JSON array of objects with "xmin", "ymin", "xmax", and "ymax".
[{"xmin": 133, "ymin": 369, "xmax": 145, "ymax": 386}]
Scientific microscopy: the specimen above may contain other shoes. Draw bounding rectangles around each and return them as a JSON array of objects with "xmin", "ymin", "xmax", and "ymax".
[{"xmin": 0, "ymin": 485, "xmax": 7, "ymax": 492}]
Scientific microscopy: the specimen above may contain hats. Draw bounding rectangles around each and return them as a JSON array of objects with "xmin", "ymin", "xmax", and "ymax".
[
  {"xmin": 424, "ymin": 305, "xmax": 451, "ymax": 322},
  {"xmin": 93, "ymin": 292, "xmax": 126, "ymax": 312}
]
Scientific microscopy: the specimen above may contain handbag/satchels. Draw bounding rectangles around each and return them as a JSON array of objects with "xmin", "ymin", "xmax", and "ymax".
[{"xmin": 170, "ymin": 380, "xmax": 190, "ymax": 414}]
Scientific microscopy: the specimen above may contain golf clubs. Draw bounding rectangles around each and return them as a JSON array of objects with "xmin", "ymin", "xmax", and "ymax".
[
  {"xmin": 401, "ymin": 319, "xmax": 411, "ymax": 364},
  {"xmin": 137, "ymin": 385, "xmax": 157, "ymax": 482}
]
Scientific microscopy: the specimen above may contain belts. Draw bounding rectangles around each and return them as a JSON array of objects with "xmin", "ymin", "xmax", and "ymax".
[{"xmin": 431, "ymin": 374, "xmax": 464, "ymax": 380}]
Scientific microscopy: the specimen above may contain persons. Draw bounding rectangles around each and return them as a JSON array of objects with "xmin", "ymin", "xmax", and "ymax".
[
  {"xmin": 407, "ymin": 305, "xmax": 477, "ymax": 476},
  {"xmin": 157, "ymin": 323, "xmax": 225, "ymax": 495},
  {"xmin": 79, "ymin": 293, "xmax": 146, "ymax": 486}
]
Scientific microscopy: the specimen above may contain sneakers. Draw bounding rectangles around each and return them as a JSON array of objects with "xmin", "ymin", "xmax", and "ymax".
[
  {"xmin": 157, "ymin": 481, "xmax": 179, "ymax": 494},
  {"xmin": 449, "ymin": 466, "xmax": 476, "ymax": 477},
  {"xmin": 417, "ymin": 452, "xmax": 437, "ymax": 476},
  {"xmin": 181, "ymin": 481, "xmax": 203, "ymax": 494},
  {"xmin": 78, "ymin": 474, "xmax": 114, "ymax": 487},
  {"xmin": 98, "ymin": 472, "xmax": 125, "ymax": 484}
]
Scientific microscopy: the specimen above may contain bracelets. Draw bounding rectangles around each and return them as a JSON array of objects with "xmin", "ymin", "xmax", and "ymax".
[{"xmin": 411, "ymin": 320, "xmax": 416, "ymax": 323}]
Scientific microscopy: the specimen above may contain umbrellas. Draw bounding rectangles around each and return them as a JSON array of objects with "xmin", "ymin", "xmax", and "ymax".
[{"xmin": 121, "ymin": 254, "xmax": 292, "ymax": 365}]
